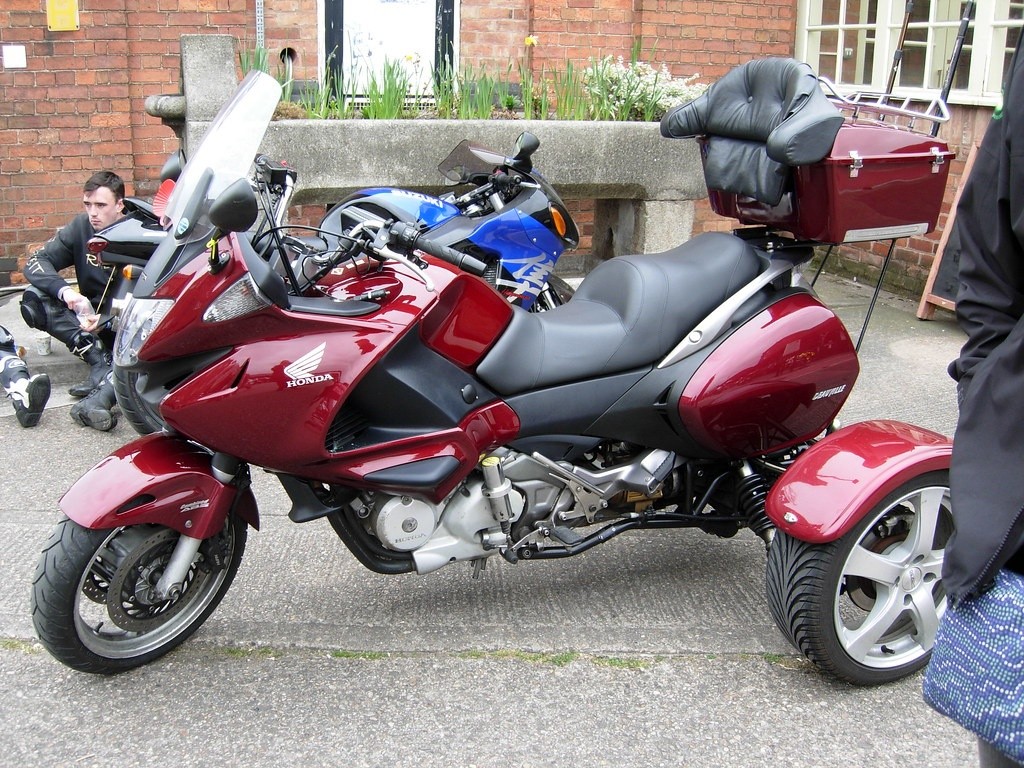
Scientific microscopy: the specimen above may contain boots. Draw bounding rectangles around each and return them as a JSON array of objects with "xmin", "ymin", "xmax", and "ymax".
[
  {"xmin": 0, "ymin": 355, "xmax": 51, "ymax": 428},
  {"xmin": 68, "ymin": 332, "xmax": 114, "ymax": 397},
  {"xmin": 69, "ymin": 369, "xmax": 118, "ymax": 431}
]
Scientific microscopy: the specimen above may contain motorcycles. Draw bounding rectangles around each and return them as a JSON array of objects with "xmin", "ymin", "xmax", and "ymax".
[
  {"xmin": 83, "ymin": 130, "xmax": 580, "ymax": 437},
  {"xmin": 29, "ymin": 57, "xmax": 952, "ymax": 689}
]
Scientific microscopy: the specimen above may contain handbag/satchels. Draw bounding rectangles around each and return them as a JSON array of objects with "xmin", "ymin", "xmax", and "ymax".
[{"xmin": 920, "ymin": 563, "xmax": 1024, "ymax": 765}]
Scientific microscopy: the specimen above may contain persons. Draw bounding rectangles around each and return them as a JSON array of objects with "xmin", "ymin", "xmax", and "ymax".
[
  {"xmin": 20, "ymin": 172, "xmax": 162, "ymax": 432},
  {"xmin": 0, "ymin": 326, "xmax": 50, "ymax": 428},
  {"xmin": 944, "ymin": 26, "xmax": 1024, "ymax": 768}
]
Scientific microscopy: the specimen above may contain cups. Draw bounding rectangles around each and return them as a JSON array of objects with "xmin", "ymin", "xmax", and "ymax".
[{"xmin": 34, "ymin": 331, "xmax": 52, "ymax": 355}]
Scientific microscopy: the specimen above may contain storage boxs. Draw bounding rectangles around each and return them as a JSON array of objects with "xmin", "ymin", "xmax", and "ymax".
[{"xmin": 695, "ymin": 120, "xmax": 956, "ymax": 245}]
